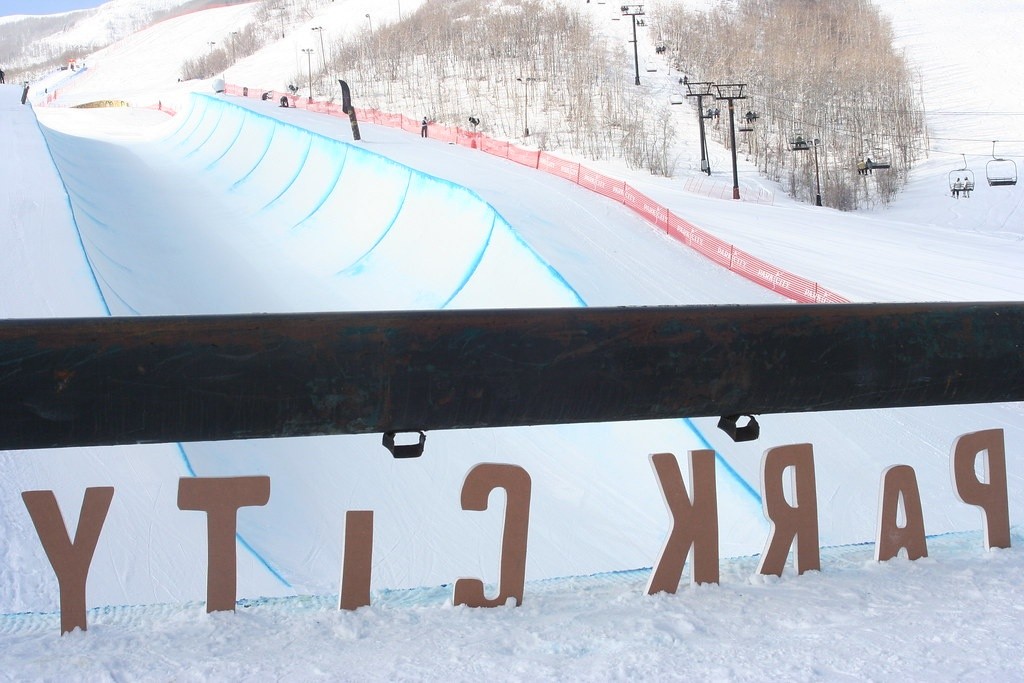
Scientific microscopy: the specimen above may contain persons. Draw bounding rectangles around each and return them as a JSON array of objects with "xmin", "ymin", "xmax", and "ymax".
[
  {"xmin": 658, "ymin": 46, "xmax": 665, "ymax": 53},
  {"xmin": 262, "ymin": 93, "xmax": 268, "ymax": 100},
  {"xmin": 858, "ymin": 158, "xmax": 872, "ymax": 175},
  {"xmin": 707, "ymin": 109, "xmax": 712, "ymax": 117},
  {"xmin": 952, "ymin": 178, "xmax": 961, "ymax": 198},
  {"xmin": 281, "ymin": 96, "xmax": 288, "ymax": 107},
  {"xmin": 715, "ymin": 109, "xmax": 720, "ymax": 118},
  {"xmin": 964, "ymin": 177, "xmax": 970, "ymax": 197},
  {"xmin": 796, "ymin": 137, "xmax": 802, "ymax": 147},
  {"xmin": 679, "ymin": 77, "xmax": 682, "ymax": 84},
  {"xmin": 684, "ymin": 76, "xmax": 687, "ymax": 84},
  {"xmin": 0, "ymin": 69, "xmax": 5, "ymax": 83},
  {"xmin": 746, "ymin": 111, "xmax": 756, "ymax": 123},
  {"xmin": 422, "ymin": 117, "xmax": 427, "ymax": 137}
]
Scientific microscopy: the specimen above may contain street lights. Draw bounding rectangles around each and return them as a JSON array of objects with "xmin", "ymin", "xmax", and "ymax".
[
  {"xmin": 232, "ymin": 32, "xmax": 237, "ymax": 64},
  {"xmin": 302, "ymin": 48, "xmax": 314, "ymax": 103},
  {"xmin": 210, "ymin": 42, "xmax": 216, "ymax": 78},
  {"xmin": 312, "ymin": 27, "xmax": 326, "ymax": 68},
  {"xmin": 281, "ymin": 7, "xmax": 286, "ymax": 38}
]
